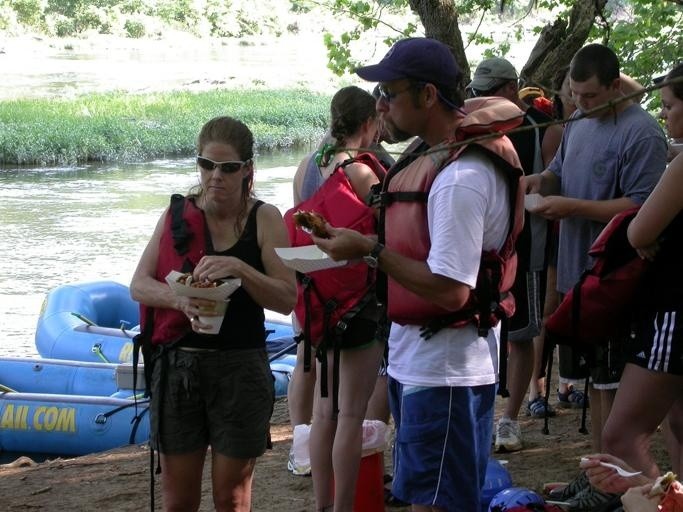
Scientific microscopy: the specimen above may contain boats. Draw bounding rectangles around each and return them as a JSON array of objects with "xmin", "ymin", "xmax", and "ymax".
[
  {"xmin": 0, "ymin": 356, "xmax": 165, "ymax": 455},
  {"xmin": 35, "ymin": 282, "xmax": 299, "ymax": 398}
]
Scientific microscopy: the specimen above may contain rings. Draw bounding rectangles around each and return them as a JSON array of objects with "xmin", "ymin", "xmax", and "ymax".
[{"xmin": 189, "ymin": 317, "xmax": 195, "ymax": 323}]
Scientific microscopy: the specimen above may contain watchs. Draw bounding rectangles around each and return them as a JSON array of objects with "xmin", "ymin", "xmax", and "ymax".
[{"xmin": 364, "ymin": 242, "xmax": 384, "ymax": 268}]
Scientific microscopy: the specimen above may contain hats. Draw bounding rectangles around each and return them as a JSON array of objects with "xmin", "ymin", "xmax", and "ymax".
[
  {"xmin": 352, "ymin": 36, "xmax": 471, "ymax": 118},
  {"xmin": 516, "ymin": 80, "xmax": 545, "ymax": 102},
  {"xmin": 464, "ymin": 57, "xmax": 521, "ymax": 94}
]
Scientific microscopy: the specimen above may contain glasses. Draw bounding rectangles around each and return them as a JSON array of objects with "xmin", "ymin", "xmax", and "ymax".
[
  {"xmin": 472, "ymin": 86, "xmax": 507, "ymax": 98},
  {"xmin": 375, "ymin": 83, "xmax": 414, "ymax": 103},
  {"xmin": 192, "ymin": 151, "xmax": 255, "ymax": 175}
]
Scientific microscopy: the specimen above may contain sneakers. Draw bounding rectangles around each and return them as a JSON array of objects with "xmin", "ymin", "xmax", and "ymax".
[
  {"xmin": 561, "ymin": 484, "xmax": 628, "ymax": 512},
  {"xmin": 281, "ymin": 456, "xmax": 313, "ymax": 481},
  {"xmin": 523, "ymin": 397, "xmax": 550, "ymax": 420},
  {"xmin": 493, "ymin": 414, "xmax": 523, "ymax": 454},
  {"xmin": 554, "ymin": 384, "xmax": 587, "ymax": 410},
  {"xmin": 548, "ymin": 474, "xmax": 591, "ymax": 501},
  {"xmin": 541, "ymin": 394, "xmax": 561, "ymax": 418}
]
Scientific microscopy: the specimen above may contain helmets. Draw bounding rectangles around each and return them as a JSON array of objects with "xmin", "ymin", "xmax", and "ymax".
[
  {"xmin": 481, "ymin": 456, "xmax": 515, "ymax": 506},
  {"xmin": 487, "ymin": 485, "xmax": 547, "ymax": 512}
]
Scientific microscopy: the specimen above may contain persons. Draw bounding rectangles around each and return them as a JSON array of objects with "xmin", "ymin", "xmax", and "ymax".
[
  {"xmin": 294, "ymin": 86, "xmax": 382, "ymax": 511},
  {"xmin": 287, "ymin": 85, "xmax": 394, "ymax": 477},
  {"xmin": 355, "ymin": 39, "xmax": 525, "ymax": 511},
  {"xmin": 578, "ymin": 453, "xmax": 683, "ymax": 512},
  {"xmin": 464, "ymin": 58, "xmax": 542, "ymax": 454},
  {"xmin": 525, "ymin": 66, "xmax": 646, "ymax": 421},
  {"xmin": 519, "ymin": 44, "xmax": 667, "ymax": 512},
  {"xmin": 599, "ymin": 61, "xmax": 683, "ymax": 482},
  {"xmin": 129, "ymin": 116, "xmax": 297, "ymax": 512}
]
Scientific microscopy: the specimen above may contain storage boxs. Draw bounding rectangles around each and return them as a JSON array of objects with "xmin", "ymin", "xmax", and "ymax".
[{"xmin": 113, "ymin": 362, "xmax": 146, "ymax": 390}]
